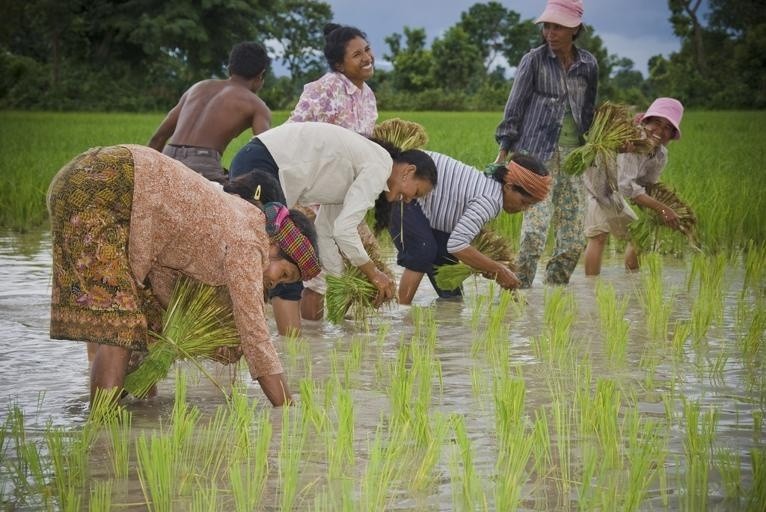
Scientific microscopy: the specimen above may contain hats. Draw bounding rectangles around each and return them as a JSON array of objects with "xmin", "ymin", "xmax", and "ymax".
[
  {"xmin": 642, "ymin": 97, "xmax": 684, "ymax": 139},
  {"xmin": 535, "ymin": 0, "xmax": 583, "ymax": 28}
]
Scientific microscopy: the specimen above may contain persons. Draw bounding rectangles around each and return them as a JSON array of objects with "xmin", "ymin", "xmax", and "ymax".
[
  {"xmin": 224, "ymin": 122, "xmax": 438, "ymax": 338},
  {"xmin": 41, "ymin": 143, "xmax": 325, "ymax": 411},
  {"xmin": 213, "ymin": 168, "xmax": 291, "ymax": 213},
  {"xmin": 584, "ymin": 98, "xmax": 684, "ymax": 278},
  {"xmin": 285, "ymin": 21, "xmax": 379, "ymax": 263},
  {"xmin": 485, "ymin": 0, "xmax": 601, "ymax": 291},
  {"xmin": 381, "ymin": 143, "xmax": 553, "ymax": 307},
  {"xmin": 141, "ymin": 37, "xmax": 275, "ymax": 178}
]
